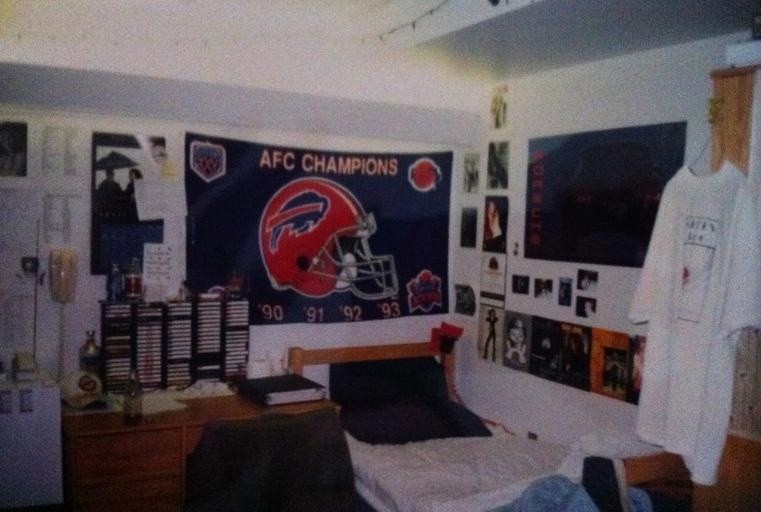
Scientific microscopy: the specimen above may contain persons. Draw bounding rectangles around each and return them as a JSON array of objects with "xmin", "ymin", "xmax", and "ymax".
[
  {"xmin": 483, "ymin": 309, "xmax": 498, "ymax": 361},
  {"xmin": 602, "ymin": 335, "xmax": 645, "ymax": 406},
  {"xmin": 98, "ymin": 168, "xmax": 122, "ymax": 192},
  {"xmin": 125, "ymin": 169, "xmax": 142, "ymax": 195},
  {"xmin": 487, "ymin": 201, "xmax": 503, "ymax": 239},
  {"xmin": 559, "ymin": 332, "xmax": 590, "ymax": 393}
]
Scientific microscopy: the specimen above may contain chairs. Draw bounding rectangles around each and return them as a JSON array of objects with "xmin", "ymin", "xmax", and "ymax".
[{"xmin": 178, "ymin": 406, "xmax": 361, "ymax": 511}]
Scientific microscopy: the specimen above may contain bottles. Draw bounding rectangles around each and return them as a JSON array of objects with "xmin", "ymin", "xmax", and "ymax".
[
  {"xmin": 124, "ymin": 369, "xmax": 143, "ymax": 426},
  {"xmin": 105, "ymin": 253, "xmax": 144, "ymax": 300},
  {"xmin": 78, "ymin": 326, "xmax": 101, "ymax": 369}
]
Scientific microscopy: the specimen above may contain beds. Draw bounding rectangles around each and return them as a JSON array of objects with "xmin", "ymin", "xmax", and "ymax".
[{"xmin": 286, "ymin": 338, "xmax": 693, "ymax": 512}]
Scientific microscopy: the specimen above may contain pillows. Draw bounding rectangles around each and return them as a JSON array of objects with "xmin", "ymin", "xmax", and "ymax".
[{"xmin": 328, "ymin": 356, "xmax": 494, "ymax": 448}]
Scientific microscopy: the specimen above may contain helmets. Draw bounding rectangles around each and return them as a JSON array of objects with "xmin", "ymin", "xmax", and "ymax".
[{"xmin": 260, "ymin": 177, "xmax": 397, "ymax": 300}]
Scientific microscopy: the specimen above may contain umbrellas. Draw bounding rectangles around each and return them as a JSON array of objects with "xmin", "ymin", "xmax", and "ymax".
[{"xmin": 93, "ymin": 151, "xmax": 139, "ymax": 170}]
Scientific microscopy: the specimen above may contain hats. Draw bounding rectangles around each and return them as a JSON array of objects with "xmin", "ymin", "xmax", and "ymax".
[{"xmin": 61, "ymin": 372, "xmax": 105, "ymax": 409}]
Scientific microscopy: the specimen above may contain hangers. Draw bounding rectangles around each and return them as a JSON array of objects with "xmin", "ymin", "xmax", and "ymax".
[{"xmin": 688, "ymin": 115, "xmax": 726, "ymax": 169}]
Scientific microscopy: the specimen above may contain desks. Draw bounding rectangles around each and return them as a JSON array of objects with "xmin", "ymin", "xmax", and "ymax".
[{"xmin": 60, "ymin": 384, "xmax": 342, "ymax": 512}]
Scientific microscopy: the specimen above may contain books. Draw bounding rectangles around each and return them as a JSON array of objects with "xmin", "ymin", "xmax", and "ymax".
[
  {"xmin": 104, "ymin": 298, "xmax": 250, "ymax": 394},
  {"xmin": 237, "ymin": 374, "xmax": 327, "ymax": 407}
]
